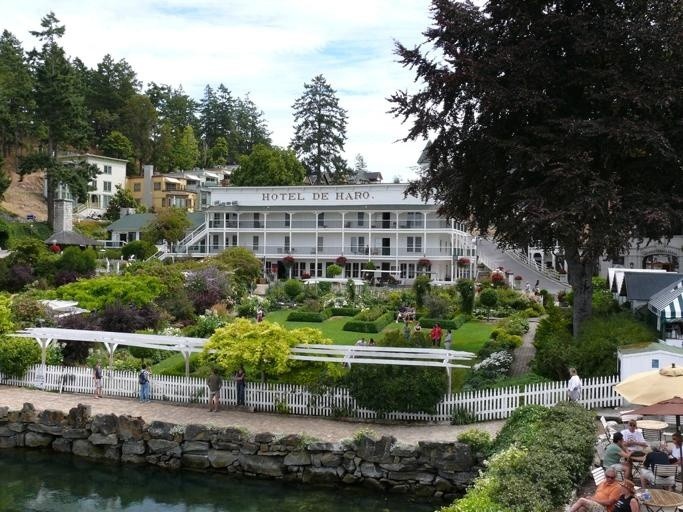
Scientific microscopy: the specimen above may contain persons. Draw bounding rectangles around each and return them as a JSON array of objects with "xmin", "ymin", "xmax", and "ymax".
[
  {"xmin": 603, "ymin": 432, "xmax": 632, "ymax": 479},
  {"xmin": 367, "ymin": 276, "xmax": 388, "ymax": 288},
  {"xmin": 639, "ymin": 441, "xmax": 674, "ymax": 492},
  {"xmin": 233, "ymin": 363, "xmax": 245, "ymax": 407},
  {"xmin": 566, "ymin": 367, "xmax": 582, "ymax": 402},
  {"xmin": 206, "ymin": 369, "xmax": 223, "ymax": 413},
  {"xmin": 666, "ymin": 429, "xmax": 683, "ymax": 466},
  {"xmin": 524, "ymin": 281, "xmax": 539, "ymax": 295},
  {"xmin": 354, "ymin": 304, "xmax": 453, "ymax": 348},
  {"xmin": 611, "ymin": 479, "xmax": 640, "ymax": 512},
  {"xmin": 620, "ymin": 419, "xmax": 648, "ymax": 463},
  {"xmin": 569, "ymin": 466, "xmax": 620, "ymax": 512},
  {"xmin": 93, "ymin": 362, "xmax": 103, "ymax": 399},
  {"xmin": 222, "ymin": 294, "xmax": 287, "ymax": 322},
  {"xmin": 138, "ymin": 364, "xmax": 152, "ymax": 404}
]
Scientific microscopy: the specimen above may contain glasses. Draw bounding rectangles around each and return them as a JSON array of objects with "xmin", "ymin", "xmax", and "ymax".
[
  {"xmin": 605, "ymin": 474, "xmax": 615, "ymax": 480},
  {"xmin": 629, "ymin": 423, "xmax": 636, "ymax": 428}
]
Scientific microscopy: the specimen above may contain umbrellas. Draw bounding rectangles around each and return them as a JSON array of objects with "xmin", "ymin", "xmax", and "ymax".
[
  {"xmin": 619, "ymin": 395, "xmax": 683, "ymax": 431},
  {"xmin": 612, "ymin": 362, "xmax": 683, "ymax": 405}
]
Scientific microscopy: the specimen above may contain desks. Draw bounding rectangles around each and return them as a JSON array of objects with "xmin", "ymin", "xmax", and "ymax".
[{"xmin": 635, "ymin": 489, "xmax": 683, "ymax": 512}]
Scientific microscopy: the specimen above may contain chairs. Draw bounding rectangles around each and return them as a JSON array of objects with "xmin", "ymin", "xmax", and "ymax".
[{"xmin": 591, "ymin": 414, "xmax": 683, "ymax": 488}]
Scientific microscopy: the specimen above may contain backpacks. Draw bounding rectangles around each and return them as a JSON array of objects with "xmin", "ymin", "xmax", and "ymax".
[{"xmin": 138, "ymin": 371, "xmax": 148, "ymax": 385}]
